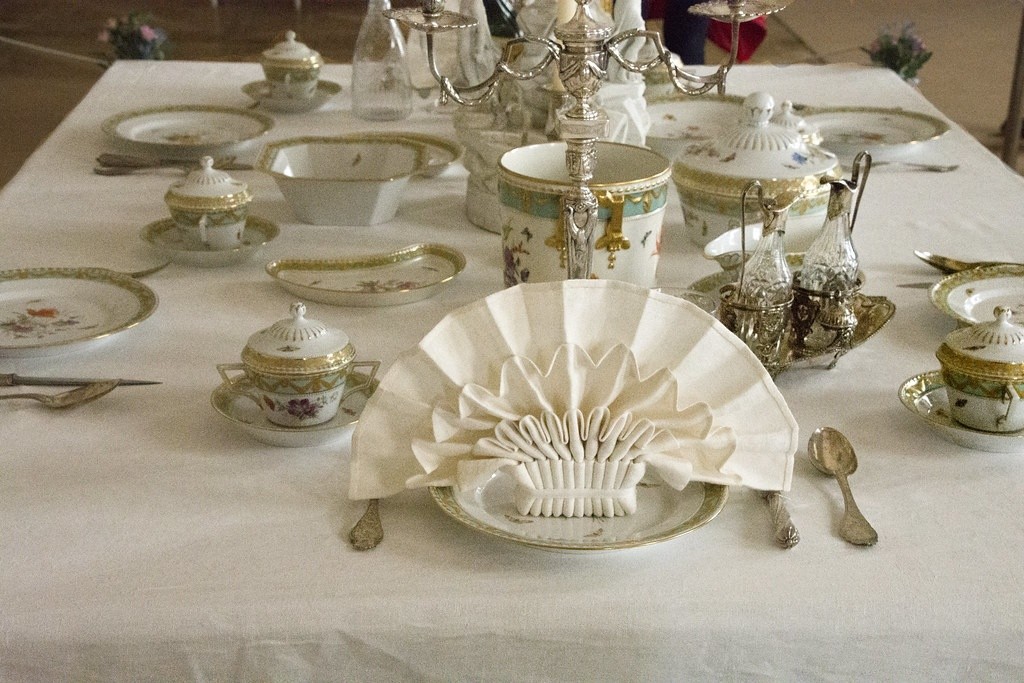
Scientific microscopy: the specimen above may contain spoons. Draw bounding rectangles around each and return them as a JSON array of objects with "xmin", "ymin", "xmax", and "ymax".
[
  {"xmin": 914, "ymin": 249, "xmax": 1024, "ymax": 274},
  {"xmin": 808, "ymin": 427, "xmax": 879, "ymax": 545},
  {"xmin": 0, "ymin": 378, "xmax": 120, "ymax": 409}
]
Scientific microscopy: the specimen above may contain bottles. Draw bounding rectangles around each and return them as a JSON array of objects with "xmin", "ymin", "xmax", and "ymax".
[{"xmin": 351, "ymin": 1, "xmax": 412, "ymax": 122}]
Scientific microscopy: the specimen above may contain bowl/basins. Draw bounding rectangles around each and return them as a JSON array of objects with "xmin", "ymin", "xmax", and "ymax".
[{"xmin": 254, "ymin": 137, "xmax": 429, "ymax": 227}]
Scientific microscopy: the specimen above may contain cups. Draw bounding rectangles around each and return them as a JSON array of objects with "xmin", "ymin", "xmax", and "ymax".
[
  {"xmin": 164, "ymin": 156, "xmax": 255, "ymax": 250},
  {"xmin": 640, "ymin": 36, "xmax": 684, "ymax": 106},
  {"xmin": 932, "ymin": 305, "xmax": 1024, "ymax": 430},
  {"xmin": 651, "ymin": 286, "xmax": 718, "ymax": 323},
  {"xmin": 672, "ymin": 93, "xmax": 843, "ymax": 248},
  {"xmin": 499, "ymin": 141, "xmax": 671, "ymax": 289},
  {"xmin": 215, "ymin": 302, "xmax": 380, "ymax": 426},
  {"xmin": 260, "ymin": 30, "xmax": 324, "ymax": 101}
]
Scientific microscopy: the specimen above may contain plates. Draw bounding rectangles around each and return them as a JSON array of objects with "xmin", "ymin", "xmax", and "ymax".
[
  {"xmin": 637, "ymin": 93, "xmax": 952, "ymax": 166},
  {"xmin": 211, "ymin": 372, "xmax": 381, "ymax": 447},
  {"xmin": 430, "ymin": 477, "xmax": 731, "ymax": 550},
  {"xmin": 101, "ymin": 80, "xmax": 464, "ymax": 178},
  {"xmin": 682, "ymin": 271, "xmax": 896, "ymax": 369},
  {"xmin": 703, "ymin": 210, "xmax": 827, "ymax": 271},
  {"xmin": 928, "ymin": 265, "xmax": 1024, "ymax": 326},
  {"xmin": 898, "ymin": 371, "xmax": 1024, "ymax": 453},
  {"xmin": 0, "ymin": 218, "xmax": 465, "ymax": 358}
]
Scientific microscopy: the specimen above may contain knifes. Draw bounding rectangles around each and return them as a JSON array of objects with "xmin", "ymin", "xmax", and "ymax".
[
  {"xmin": 0, "ymin": 372, "xmax": 163, "ymax": 387},
  {"xmin": 120, "ymin": 260, "xmax": 173, "ymax": 278},
  {"xmin": 97, "ymin": 153, "xmax": 254, "ymax": 171}
]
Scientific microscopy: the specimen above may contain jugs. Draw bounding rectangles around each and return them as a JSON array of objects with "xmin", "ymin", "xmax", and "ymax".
[{"xmin": 718, "ymin": 151, "xmax": 872, "ymax": 385}]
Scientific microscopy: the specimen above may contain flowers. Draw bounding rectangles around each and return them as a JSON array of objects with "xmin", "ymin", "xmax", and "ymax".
[
  {"xmin": 860, "ymin": 22, "xmax": 935, "ymax": 84},
  {"xmin": 97, "ymin": 11, "xmax": 170, "ymax": 70}
]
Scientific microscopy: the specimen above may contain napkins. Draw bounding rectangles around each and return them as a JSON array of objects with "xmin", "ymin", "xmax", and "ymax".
[
  {"xmin": 350, "ymin": 280, "xmax": 799, "ymax": 516},
  {"xmin": 599, "ymin": 81, "xmax": 650, "ymax": 147},
  {"xmin": 406, "ymin": 0, "xmax": 502, "ymax": 90}
]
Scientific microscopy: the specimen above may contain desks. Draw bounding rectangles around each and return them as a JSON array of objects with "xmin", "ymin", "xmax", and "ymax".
[{"xmin": 0, "ymin": 60, "xmax": 1024, "ymax": 683}]
